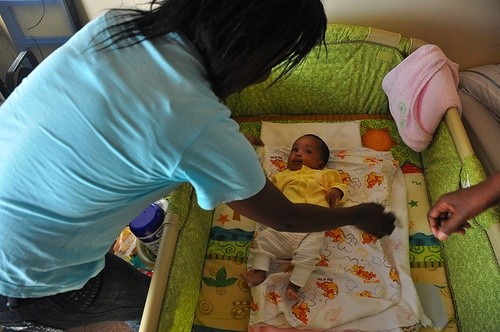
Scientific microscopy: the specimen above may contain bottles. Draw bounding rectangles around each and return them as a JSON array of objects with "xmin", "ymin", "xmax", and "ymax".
[{"xmin": 129, "ymin": 202, "xmax": 165, "ymax": 261}]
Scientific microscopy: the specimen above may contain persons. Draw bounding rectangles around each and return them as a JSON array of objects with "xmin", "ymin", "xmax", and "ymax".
[
  {"xmin": 240, "ymin": 134, "xmax": 347, "ymax": 299},
  {"xmin": 427, "ymin": 172, "xmax": 500, "ymax": 244},
  {"xmin": 0, "ymin": 0, "xmax": 396, "ymax": 332}
]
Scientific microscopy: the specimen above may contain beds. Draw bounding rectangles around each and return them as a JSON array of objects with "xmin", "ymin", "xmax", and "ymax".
[{"xmin": 136, "ymin": 24, "xmax": 500, "ymax": 332}]
